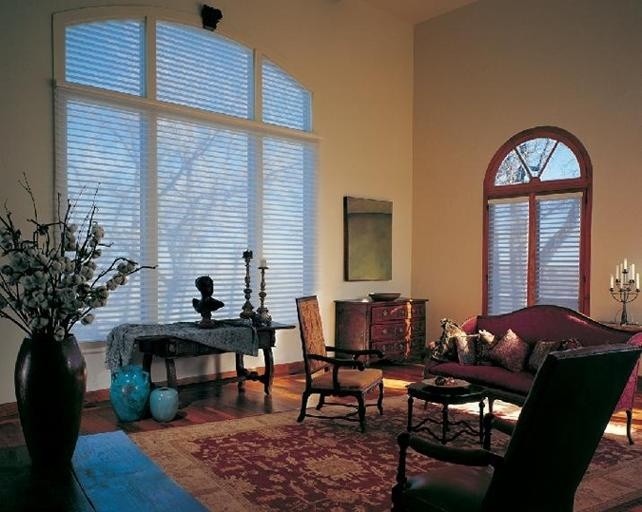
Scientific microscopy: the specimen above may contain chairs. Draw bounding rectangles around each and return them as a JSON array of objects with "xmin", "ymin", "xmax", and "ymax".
[
  {"xmin": 295, "ymin": 295, "xmax": 385, "ymax": 433},
  {"xmin": 390, "ymin": 344, "xmax": 641, "ymax": 510}
]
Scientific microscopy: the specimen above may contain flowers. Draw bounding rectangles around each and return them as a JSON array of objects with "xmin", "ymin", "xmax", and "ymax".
[{"xmin": 0, "ymin": 169, "xmax": 159, "ymax": 339}]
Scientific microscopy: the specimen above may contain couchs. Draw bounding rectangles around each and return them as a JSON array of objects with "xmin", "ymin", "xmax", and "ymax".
[{"xmin": 424, "ymin": 304, "xmax": 642, "ymax": 446}]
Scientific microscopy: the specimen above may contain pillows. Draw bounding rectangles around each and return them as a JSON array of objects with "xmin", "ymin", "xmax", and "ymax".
[{"xmin": 430, "ymin": 316, "xmax": 585, "ymax": 376}]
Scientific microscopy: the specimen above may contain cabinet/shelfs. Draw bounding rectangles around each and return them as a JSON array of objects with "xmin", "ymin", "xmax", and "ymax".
[{"xmin": 333, "ymin": 294, "xmax": 430, "ymax": 367}]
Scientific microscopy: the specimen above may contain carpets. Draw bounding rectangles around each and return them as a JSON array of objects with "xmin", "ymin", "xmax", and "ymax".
[{"xmin": 125, "ymin": 396, "xmax": 642, "ymax": 511}]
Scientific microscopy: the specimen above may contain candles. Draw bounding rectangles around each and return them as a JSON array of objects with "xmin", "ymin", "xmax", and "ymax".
[
  {"xmin": 257, "ymin": 257, "xmax": 268, "ymax": 269},
  {"xmin": 609, "ymin": 258, "xmax": 640, "ymax": 293},
  {"xmin": 242, "ymin": 248, "xmax": 253, "ymax": 259}
]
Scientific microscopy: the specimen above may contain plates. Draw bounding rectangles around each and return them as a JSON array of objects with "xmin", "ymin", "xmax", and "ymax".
[{"xmin": 422, "ymin": 377, "xmax": 471, "ymax": 387}]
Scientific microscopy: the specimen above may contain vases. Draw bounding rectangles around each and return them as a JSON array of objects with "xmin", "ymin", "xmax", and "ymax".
[
  {"xmin": 15, "ymin": 334, "xmax": 87, "ymax": 472},
  {"xmin": 109, "ymin": 362, "xmax": 152, "ymax": 423},
  {"xmin": 150, "ymin": 384, "xmax": 179, "ymax": 423}
]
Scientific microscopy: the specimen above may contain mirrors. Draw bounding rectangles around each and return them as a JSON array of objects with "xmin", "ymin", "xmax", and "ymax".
[{"xmin": 343, "ymin": 195, "xmax": 394, "ymax": 282}]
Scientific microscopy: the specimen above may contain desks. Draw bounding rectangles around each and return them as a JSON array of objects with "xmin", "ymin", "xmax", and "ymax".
[
  {"xmin": 137, "ymin": 315, "xmax": 296, "ymax": 409},
  {"xmin": 1, "ymin": 430, "xmax": 210, "ymax": 512},
  {"xmin": 598, "ymin": 321, "xmax": 642, "ymax": 334}
]
